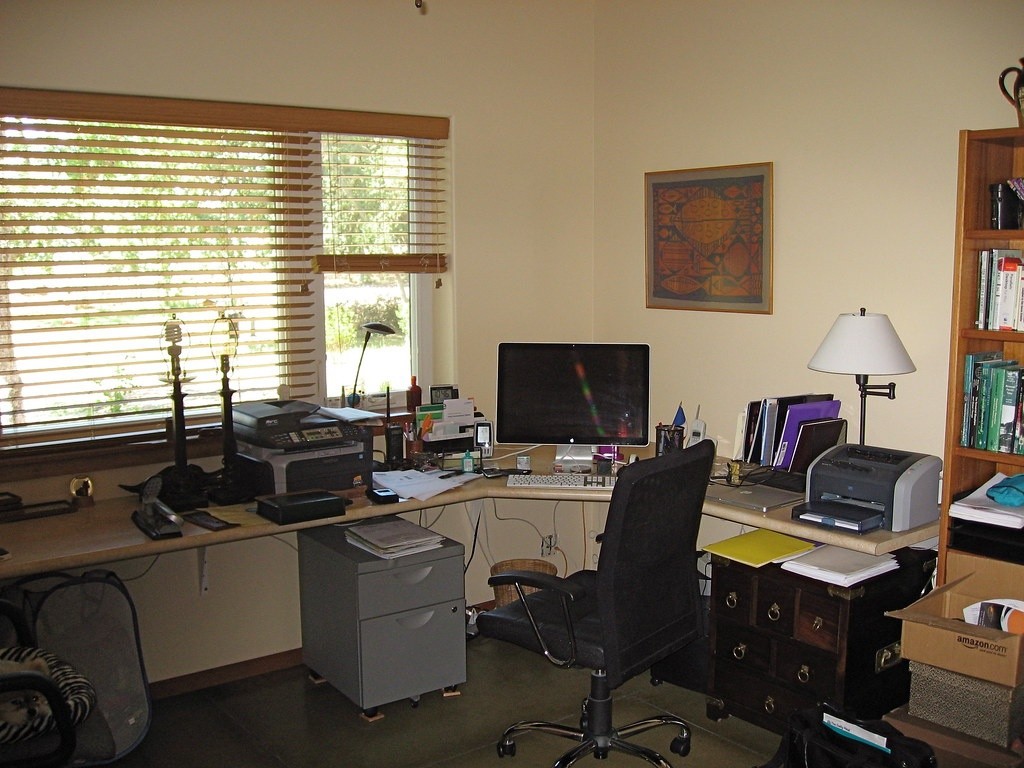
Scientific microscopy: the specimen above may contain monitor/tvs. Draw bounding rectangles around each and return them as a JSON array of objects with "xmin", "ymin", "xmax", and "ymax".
[{"xmin": 495, "ymin": 342, "xmax": 650, "ymax": 474}]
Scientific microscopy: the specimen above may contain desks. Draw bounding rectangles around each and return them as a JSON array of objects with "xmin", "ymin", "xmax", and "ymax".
[{"xmin": 0, "ymin": 477, "xmax": 941, "ymax": 767}]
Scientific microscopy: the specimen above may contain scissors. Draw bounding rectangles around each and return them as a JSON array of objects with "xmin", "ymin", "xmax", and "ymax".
[{"xmin": 421, "ymin": 413, "xmax": 434, "ymax": 438}]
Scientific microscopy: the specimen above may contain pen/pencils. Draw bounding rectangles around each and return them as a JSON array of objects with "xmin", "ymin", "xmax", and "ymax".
[
  {"xmin": 658, "ymin": 422, "xmax": 680, "ymax": 457},
  {"xmin": 438, "ymin": 470, "xmax": 463, "ymax": 479},
  {"xmin": 669, "ymin": 401, "xmax": 682, "ymax": 437}
]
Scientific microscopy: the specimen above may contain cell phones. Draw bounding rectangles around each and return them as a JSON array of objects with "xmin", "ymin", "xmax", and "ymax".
[
  {"xmin": 481, "ymin": 469, "xmax": 502, "ymax": 477},
  {"xmin": 474, "ymin": 421, "xmax": 494, "ymax": 457}
]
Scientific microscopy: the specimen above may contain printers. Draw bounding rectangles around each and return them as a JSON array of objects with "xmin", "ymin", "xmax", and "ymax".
[
  {"xmin": 791, "ymin": 443, "xmax": 944, "ymax": 536},
  {"xmin": 232, "ymin": 400, "xmax": 373, "ymax": 501}
]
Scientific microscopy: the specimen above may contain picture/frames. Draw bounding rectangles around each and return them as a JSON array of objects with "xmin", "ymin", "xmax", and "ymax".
[{"xmin": 645, "ymin": 161, "xmax": 775, "ymax": 316}]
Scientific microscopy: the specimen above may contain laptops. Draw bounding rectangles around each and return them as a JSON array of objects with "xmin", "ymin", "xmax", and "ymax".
[{"xmin": 704, "ymin": 480, "xmax": 805, "ymax": 513}]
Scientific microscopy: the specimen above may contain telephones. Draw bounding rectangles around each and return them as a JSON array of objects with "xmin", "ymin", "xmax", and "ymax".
[{"xmin": 685, "ymin": 404, "xmax": 718, "ymax": 456}]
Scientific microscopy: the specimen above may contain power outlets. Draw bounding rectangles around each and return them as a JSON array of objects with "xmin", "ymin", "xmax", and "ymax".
[{"xmin": 542, "ymin": 533, "xmax": 560, "ymax": 557}]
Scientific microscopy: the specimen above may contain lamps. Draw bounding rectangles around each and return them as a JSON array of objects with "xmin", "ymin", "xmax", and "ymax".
[
  {"xmin": 809, "ymin": 308, "xmax": 916, "ymax": 447},
  {"xmin": 161, "ymin": 311, "xmax": 257, "ymax": 512},
  {"xmin": 350, "ymin": 321, "xmax": 396, "ymax": 407}
]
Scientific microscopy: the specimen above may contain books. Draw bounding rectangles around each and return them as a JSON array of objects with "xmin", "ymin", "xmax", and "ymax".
[
  {"xmin": 958, "ymin": 351, "xmax": 1024, "ymax": 456},
  {"xmin": 734, "ymin": 392, "xmax": 845, "ymax": 473},
  {"xmin": 343, "ymin": 519, "xmax": 446, "ymax": 560},
  {"xmin": 702, "ymin": 528, "xmax": 816, "ymax": 568},
  {"xmin": 1008, "ymin": 177, "xmax": 1024, "ymax": 199},
  {"xmin": 975, "ymin": 248, "xmax": 1024, "ymax": 332},
  {"xmin": 318, "ymin": 406, "xmax": 385, "ymax": 423}
]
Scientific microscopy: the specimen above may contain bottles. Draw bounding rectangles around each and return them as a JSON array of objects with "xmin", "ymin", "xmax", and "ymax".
[
  {"xmin": 406, "ymin": 376, "xmax": 422, "ymax": 412},
  {"xmin": 462, "ymin": 450, "xmax": 474, "ymax": 472}
]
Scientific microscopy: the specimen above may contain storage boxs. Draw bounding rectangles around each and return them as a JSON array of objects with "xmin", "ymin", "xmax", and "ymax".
[{"xmin": 880, "ymin": 560, "xmax": 1022, "ymax": 749}]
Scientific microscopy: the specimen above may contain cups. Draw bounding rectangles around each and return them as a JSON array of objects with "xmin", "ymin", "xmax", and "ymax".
[{"xmin": 406, "ymin": 440, "xmax": 422, "ymax": 462}]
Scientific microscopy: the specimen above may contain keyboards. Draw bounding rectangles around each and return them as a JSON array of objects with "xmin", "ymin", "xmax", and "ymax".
[{"xmin": 506, "ymin": 474, "xmax": 618, "ymax": 491}]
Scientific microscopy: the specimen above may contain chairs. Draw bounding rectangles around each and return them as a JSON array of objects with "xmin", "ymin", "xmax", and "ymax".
[
  {"xmin": 0, "ymin": 600, "xmax": 118, "ymax": 768},
  {"xmin": 476, "ymin": 438, "xmax": 717, "ymax": 767}
]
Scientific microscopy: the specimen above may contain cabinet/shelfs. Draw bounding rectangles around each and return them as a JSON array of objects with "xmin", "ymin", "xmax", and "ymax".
[
  {"xmin": 937, "ymin": 123, "xmax": 1024, "ymax": 588},
  {"xmin": 705, "ymin": 536, "xmax": 940, "ymax": 738},
  {"xmin": 298, "ymin": 515, "xmax": 468, "ymax": 721}
]
